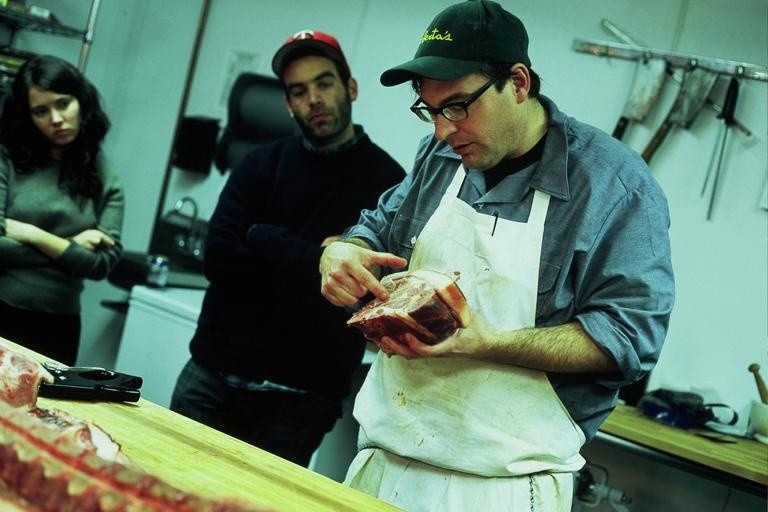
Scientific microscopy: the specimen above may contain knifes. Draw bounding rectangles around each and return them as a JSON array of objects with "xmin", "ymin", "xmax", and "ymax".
[
  {"xmin": 638, "ymin": 65, "xmax": 721, "ymax": 163},
  {"xmin": 609, "ymin": 55, "xmax": 668, "ymax": 141}
]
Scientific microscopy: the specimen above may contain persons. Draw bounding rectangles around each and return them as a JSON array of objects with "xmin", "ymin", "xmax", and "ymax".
[
  {"xmin": 1, "ymin": 54, "xmax": 125, "ymax": 369},
  {"xmin": 168, "ymin": 29, "xmax": 409, "ymax": 468},
  {"xmin": 318, "ymin": 0, "xmax": 678, "ymax": 512}
]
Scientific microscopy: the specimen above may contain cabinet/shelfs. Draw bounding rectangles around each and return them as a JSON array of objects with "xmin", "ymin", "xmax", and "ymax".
[{"xmin": 0, "ymin": 0, "xmax": 100, "ymax": 88}]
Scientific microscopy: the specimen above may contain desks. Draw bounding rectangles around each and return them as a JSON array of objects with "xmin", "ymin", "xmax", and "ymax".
[
  {"xmin": 0, "ymin": 333, "xmax": 403, "ymax": 512},
  {"xmin": 573, "ymin": 401, "xmax": 767, "ymax": 511}
]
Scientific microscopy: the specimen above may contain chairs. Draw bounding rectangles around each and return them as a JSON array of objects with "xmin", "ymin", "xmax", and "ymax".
[{"xmin": 100, "ymin": 208, "xmax": 214, "ymax": 323}]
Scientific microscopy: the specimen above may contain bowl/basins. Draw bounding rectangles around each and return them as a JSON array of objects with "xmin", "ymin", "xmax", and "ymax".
[{"xmin": 748, "ymin": 399, "xmax": 767, "ymax": 445}]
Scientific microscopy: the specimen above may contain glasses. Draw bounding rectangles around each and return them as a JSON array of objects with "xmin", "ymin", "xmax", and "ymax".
[{"xmin": 410, "ymin": 66, "xmax": 510, "ymax": 123}]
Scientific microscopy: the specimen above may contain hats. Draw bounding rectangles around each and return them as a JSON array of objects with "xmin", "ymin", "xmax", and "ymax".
[
  {"xmin": 272, "ymin": 30, "xmax": 350, "ymax": 79},
  {"xmin": 380, "ymin": 0, "xmax": 531, "ymax": 87}
]
